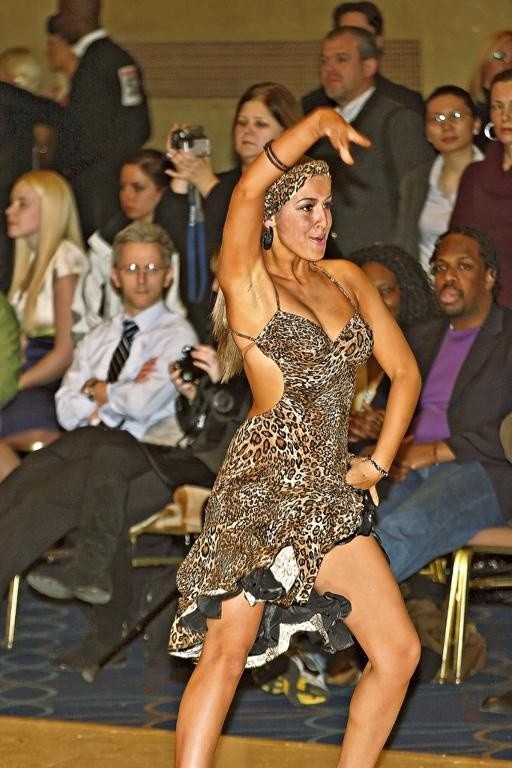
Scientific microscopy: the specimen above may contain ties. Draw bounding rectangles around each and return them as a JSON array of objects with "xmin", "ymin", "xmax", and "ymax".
[{"xmin": 107, "ymin": 321, "xmax": 137, "ymax": 382}]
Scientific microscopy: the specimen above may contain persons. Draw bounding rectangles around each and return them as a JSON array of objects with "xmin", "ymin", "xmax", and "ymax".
[
  {"xmin": 165, "ymin": 107, "xmax": 423, "ymax": 767},
  {"xmin": 1, "ymin": 1, "xmax": 512, "ymax": 685}
]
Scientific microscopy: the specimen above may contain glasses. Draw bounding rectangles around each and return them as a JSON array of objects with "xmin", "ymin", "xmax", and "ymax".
[
  {"xmin": 427, "ymin": 111, "xmax": 470, "ymax": 123},
  {"xmin": 122, "ymin": 263, "xmax": 167, "ymax": 273}
]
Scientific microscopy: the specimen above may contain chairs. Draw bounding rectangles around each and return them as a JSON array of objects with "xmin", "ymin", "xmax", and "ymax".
[
  {"xmin": 432, "ymin": 526, "xmax": 508, "ymax": 683},
  {"xmin": 4, "ymin": 492, "xmax": 203, "ymax": 652}
]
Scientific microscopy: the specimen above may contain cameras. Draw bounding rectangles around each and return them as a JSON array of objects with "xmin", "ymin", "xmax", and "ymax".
[
  {"xmin": 169, "ymin": 123, "xmax": 213, "ymax": 162},
  {"xmin": 178, "ymin": 343, "xmax": 211, "ymax": 380}
]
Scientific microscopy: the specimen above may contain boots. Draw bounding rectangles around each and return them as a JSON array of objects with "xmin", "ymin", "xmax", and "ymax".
[{"xmin": 27, "ymin": 447, "xmax": 129, "ymax": 604}]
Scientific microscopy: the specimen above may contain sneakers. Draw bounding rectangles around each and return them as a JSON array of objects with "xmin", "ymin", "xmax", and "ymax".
[{"xmin": 252, "ymin": 643, "xmax": 329, "ymax": 709}]
